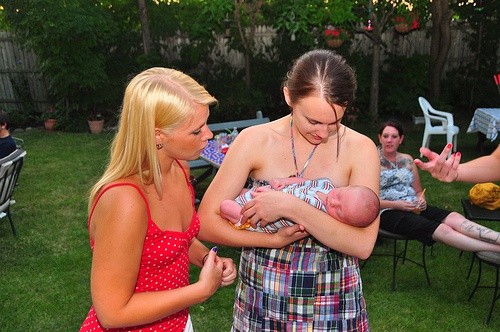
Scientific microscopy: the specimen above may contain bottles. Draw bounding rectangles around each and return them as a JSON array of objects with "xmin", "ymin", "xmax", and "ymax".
[{"xmin": 232, "ymin": 127, "xmax": 238, "ymax": 141}]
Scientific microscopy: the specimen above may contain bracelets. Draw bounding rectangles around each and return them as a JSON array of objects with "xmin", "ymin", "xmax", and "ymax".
[{"xmin": 202, "ymin": 253, "xmax": 209, "ymax": 265}]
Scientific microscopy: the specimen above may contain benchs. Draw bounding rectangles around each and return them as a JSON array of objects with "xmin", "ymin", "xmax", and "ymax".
[{"xmin": 184, "ymin": 111, "xmax": 270, "ymax": 170}]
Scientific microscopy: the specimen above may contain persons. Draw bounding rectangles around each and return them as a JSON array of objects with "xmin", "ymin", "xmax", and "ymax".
[
  {"xmin": 0, "ymin": 111, "xmax": 16, "ymax": 160},
  {"xmin": 198, "ymin": 49, "xmax": 382, "ymax": 332},
  {"xmin": 414, "ymin": 143, "xmax": 500, "ymax": 182},
  {"xmin": 80, "ymin": 67, "xmax": 237, "ymax": 332},
  {"xmin": 375, "ymin": 120, "xmax": 500, "ymax": 253},
  {"xmin": 220, "ymin": 177, "xmax": 380, "ymax": 233}
]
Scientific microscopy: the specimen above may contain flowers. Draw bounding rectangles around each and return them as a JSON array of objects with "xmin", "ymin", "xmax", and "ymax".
[
  {"xmin": 89, "ymin": 112, "xmax": 105, "ymax": 121},
  {"xmin": 322, "ymin": 24, "xmax": 351, "ymax": 40},
  {"xmin": 40, "ymin": 106, "xmax": 60, "ymax": 120},
  {"xmin": 392, "ymin": 13, "xmax": 420, "ymax": 28}
]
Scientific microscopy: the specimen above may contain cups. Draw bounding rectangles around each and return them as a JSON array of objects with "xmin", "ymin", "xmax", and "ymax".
[{"xmin": 411, "ymin": 197, "xmax": 421, "ymax": 214}]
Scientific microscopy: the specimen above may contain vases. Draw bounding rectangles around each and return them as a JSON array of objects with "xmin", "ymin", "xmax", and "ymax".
[
  {"xmin": 44, "ymin": 119, "xmax": 60, "ymax": 131},
  {"xmin": 394, "ymin": 23, "xmax": 410, "ymax": 33},
  {"xmin": 326, "ymin": 38, "xmax": 344, "ymax": 48},
  {"xmin": 88, "ymin": 119, "xmax": 104, "ymax": 134}
]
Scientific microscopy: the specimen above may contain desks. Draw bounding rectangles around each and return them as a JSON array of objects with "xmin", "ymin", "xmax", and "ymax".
[
  {"xmin": 190, "ymin": 133, "xmax": 239, "ymax": 190},
  {"xmin": 467, "ymin": 108, "xmax": 500, "ymax": 157},
  {"xmin": 458, "ymin": 198, "xmax": 500, "ymax": 256}
]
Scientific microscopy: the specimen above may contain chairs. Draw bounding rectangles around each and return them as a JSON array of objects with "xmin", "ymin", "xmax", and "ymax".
[
  {"xmin": 418, "ymin": 96, "xmax": 459, "ymax": 157},
  {"xmin": 361, "ymin": 229, "xmax": 433, "ymax": 291},
  {"xmin": 0, "ymin": 145, "xmax": 28, "ymax": 237},
  {"xmin": 463, "ymin": 248, "xmax": 500, "ymax": 326}
]
